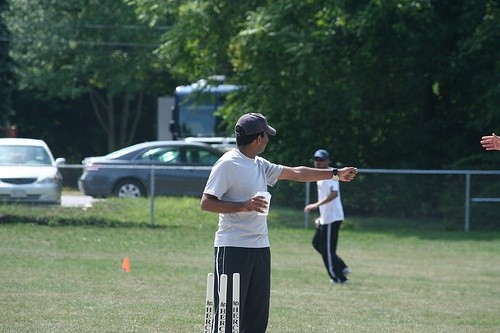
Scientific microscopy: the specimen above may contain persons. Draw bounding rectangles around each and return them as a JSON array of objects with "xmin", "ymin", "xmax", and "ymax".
[
  {"xmin": 304, "ymin": 149, "xmax": 352, "ymax": 284},
  {"xmin": 200, "ymin": 113, "xmax": 357, "ymax": 333},
  {"xmin": 480, "ymin": 133, "xmax": 500, "ymax": 151}
]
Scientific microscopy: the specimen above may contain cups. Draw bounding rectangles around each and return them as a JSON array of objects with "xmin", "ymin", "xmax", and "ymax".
[{"xmin": 256, "ymin": 192, "xmax": 271, "ymax": 216}]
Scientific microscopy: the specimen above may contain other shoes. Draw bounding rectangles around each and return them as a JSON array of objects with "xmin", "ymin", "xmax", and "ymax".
[{"xmin": 343, "ymin": 266, "xmax": 348, "ymax": 275}]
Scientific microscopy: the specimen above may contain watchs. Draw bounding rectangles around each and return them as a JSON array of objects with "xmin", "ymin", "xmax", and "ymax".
[{"xmin": 332, "ymin": 169, "xmax": 339, "ymax": 181}]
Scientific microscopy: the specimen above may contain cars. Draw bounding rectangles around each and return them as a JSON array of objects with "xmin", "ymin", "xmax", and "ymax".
[
  {"xmin": 78, "ymin": 141, "xmax": 227, "ymax": 198},
  {"xmin": 0, "ymin": 138, "xmax": 66, "ymax": 207},
  {"xmin": 143, "ymin": 137, "xmax": 237, "ymax": 164}
]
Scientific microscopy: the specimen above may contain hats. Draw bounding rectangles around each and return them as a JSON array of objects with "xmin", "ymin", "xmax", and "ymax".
[
  {"xmin": 314, "ymin": 149, "xmax": 329, "ymax": 161},
  {"xmin": 234, "ymin": 113, "xmax": 276, "ymax": 137}
]
